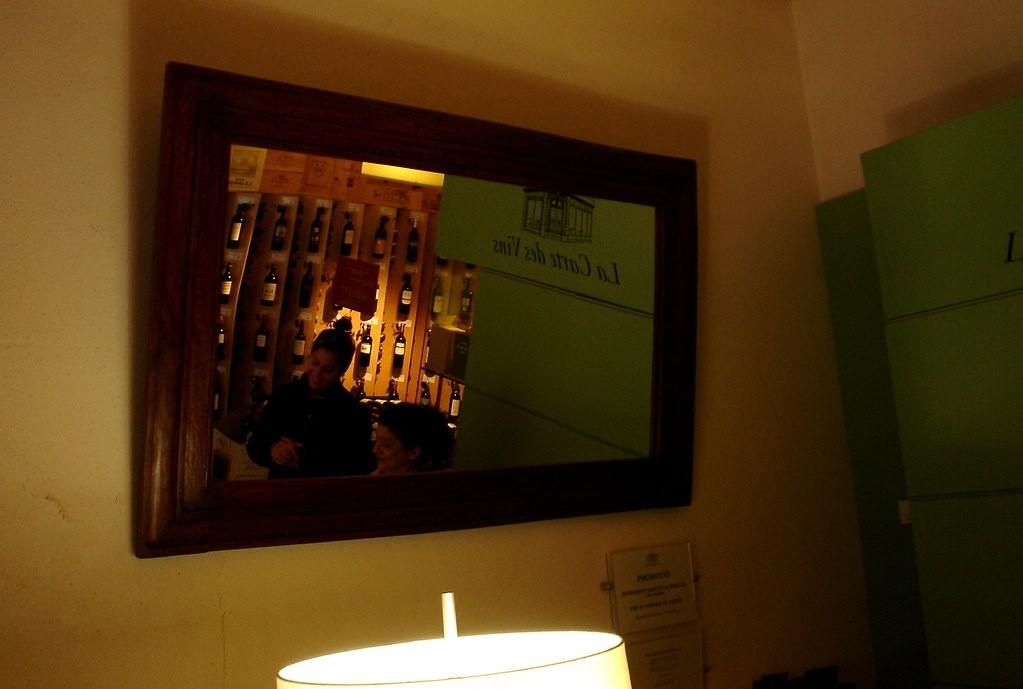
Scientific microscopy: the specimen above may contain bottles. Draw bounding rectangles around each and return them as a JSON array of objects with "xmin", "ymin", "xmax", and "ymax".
[{"xmin": 215, "ymin": 205, "xmax": 472, "ymax": 423}]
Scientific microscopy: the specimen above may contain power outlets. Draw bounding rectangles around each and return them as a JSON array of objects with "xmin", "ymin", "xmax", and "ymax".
[{"xmin": 899, "ymin": 500, "xmax": 912, "ymax": 524}]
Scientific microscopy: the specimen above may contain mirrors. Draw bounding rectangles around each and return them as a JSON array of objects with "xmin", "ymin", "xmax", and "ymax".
[{"xmin": 130, "ymin": 60, "xmax": 700, "ymax": 559}]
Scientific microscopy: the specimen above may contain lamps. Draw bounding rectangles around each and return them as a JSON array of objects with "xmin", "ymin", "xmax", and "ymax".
[{"xmin": 279, "ymin": 591, "xmax": 633, "ymax": 689}]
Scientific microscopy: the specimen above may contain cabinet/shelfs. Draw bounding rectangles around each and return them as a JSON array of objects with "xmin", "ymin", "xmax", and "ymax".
[
  {"xmin": 433, "ymin": 174, "xmax": 658, "ymax": 467},
  {"xmin": 860, "ymin": 94, "xmax": 1023, "ymax": 689}
]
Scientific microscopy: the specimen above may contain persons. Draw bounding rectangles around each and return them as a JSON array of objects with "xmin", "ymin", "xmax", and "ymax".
[
  {"xmin": 246, "ymin": 318, "xmax": 377, "ymax": 478},
  {"xmin": 370, "ymin": 404, "xmax": 448, "ymax": 474}
]
